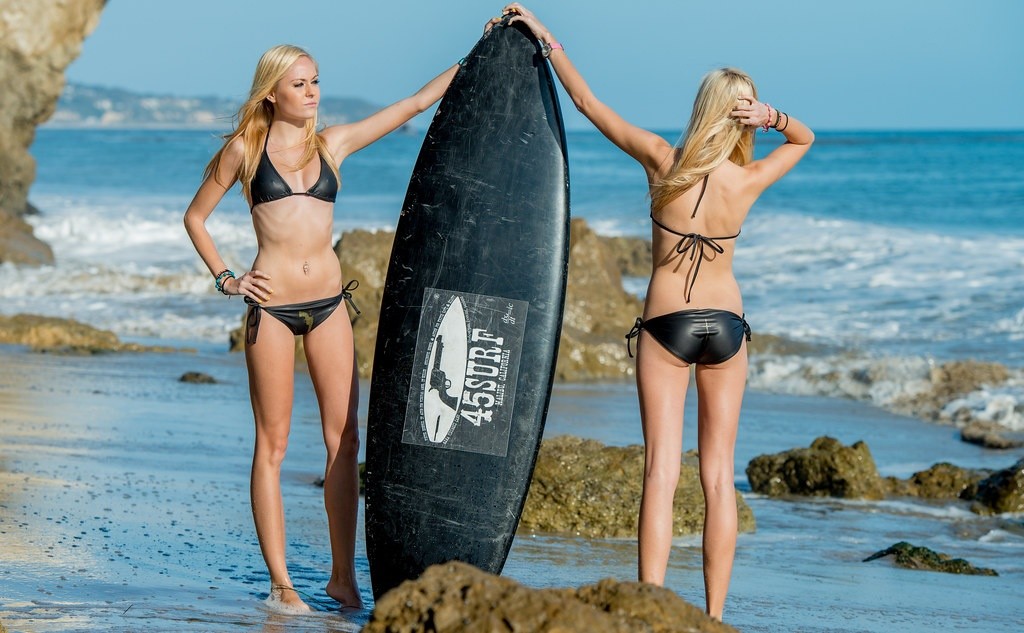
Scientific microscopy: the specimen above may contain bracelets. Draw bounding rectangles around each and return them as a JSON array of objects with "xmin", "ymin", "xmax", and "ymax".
[
  {"xmin": 213, "ymin": 269, "xmax": 234, "ymax": 300},
  {"xmin": 765, "ymin": 102, "xmax": 789, "ymax": 136}
]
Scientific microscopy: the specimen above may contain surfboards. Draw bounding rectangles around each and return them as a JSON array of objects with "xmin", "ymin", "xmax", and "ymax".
[{"xmin": 365, "ymin": 6, "xmax": 572, "ymax": 610}]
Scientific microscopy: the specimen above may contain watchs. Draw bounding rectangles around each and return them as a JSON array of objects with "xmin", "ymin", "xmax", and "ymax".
[{"xmin": 541, "ymin": 42, "xmax": 564, "ymax": 57}]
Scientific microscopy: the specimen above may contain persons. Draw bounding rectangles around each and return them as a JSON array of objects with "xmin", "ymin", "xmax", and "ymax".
[
  {"xmin": 183, "ymin": 16, "xmax": 499, "ymax": 613},
  {"xmin": 501, "ymin": 0, "xmax": 815, "ymax": 622}
]
